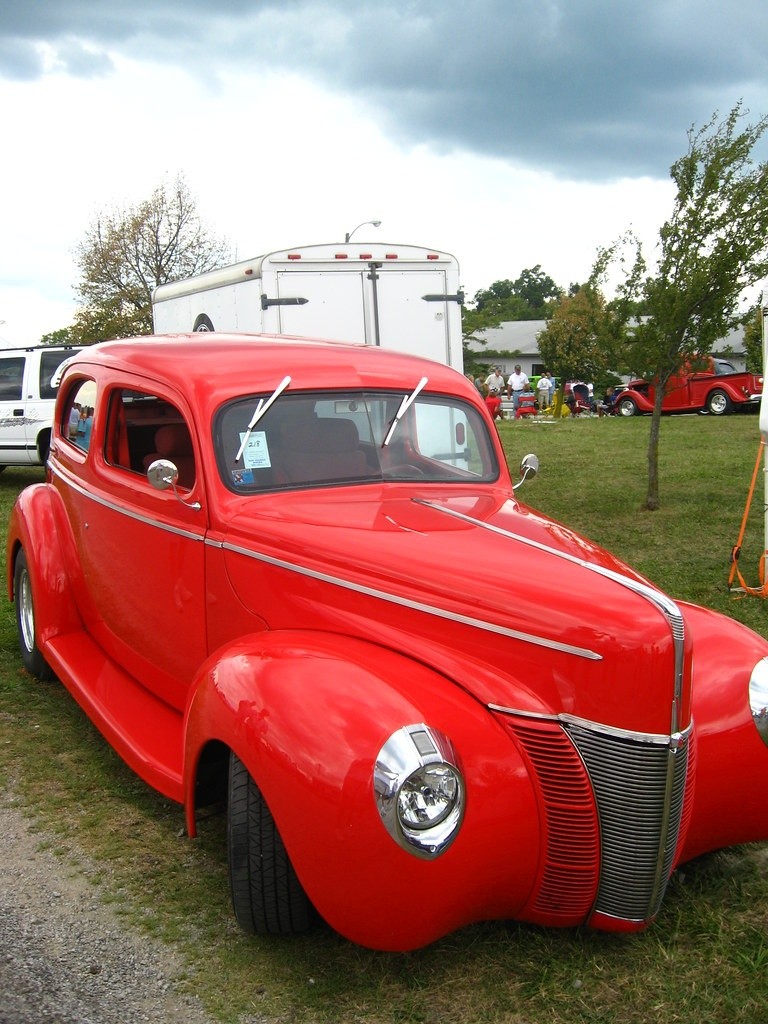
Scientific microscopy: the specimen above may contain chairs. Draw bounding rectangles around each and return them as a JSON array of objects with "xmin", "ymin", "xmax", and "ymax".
[
  {"xmin": 269, "ymin": 418, "xmax": 367, "ymax": 481},
  {"xmin": 142, "ymin": 424, "xmax": 259, "ymax": 490}
]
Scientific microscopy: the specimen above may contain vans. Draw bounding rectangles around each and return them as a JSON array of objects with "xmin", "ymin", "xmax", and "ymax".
[{"xmin": 0, "ymin": 345, "xmax": 159, "ymax": 484}]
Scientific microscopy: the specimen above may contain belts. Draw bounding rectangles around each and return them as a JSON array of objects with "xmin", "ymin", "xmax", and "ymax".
[{"xmin": 514, "ymin": 389, "xmax": 523, "ymax": 392}]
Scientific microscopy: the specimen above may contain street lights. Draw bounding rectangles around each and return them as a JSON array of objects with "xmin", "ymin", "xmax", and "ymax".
[{"xmin": 346, "ymin": 220, "xmax": 382, "ymax": 243}]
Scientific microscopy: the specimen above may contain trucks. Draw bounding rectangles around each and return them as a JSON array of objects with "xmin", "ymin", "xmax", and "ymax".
[{"xmin": 152, "ymin": 243, "xmax": 470, "ymax": 472}]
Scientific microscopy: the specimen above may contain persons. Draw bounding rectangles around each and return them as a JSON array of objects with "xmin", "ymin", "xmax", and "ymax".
[
  {"xmin": 66, "ymin": 402, "xmax": 95, "ymax": 452},
  {"xmin": 597, "ymin": 387, "xmax": 615, "ymax": 416},
  {"xmin": 474, "ymin": 364, "xmax": 555, "ymax": 420}
]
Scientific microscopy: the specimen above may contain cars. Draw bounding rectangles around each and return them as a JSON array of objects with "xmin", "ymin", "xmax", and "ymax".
[{"xmin": 7, "ymin": 334, "xmax": 768, "ymax": 953}]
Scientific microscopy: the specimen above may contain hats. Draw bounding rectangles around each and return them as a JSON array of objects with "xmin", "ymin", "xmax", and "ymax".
[{"xmin": 496, "ymin": 367, "xmax": 502, "ymax": 371}]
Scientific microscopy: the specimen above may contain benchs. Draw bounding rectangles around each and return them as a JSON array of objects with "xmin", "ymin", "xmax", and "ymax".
[{"xmin": 127, "ymin": 412, "xmax": 317, "ymax": 476}]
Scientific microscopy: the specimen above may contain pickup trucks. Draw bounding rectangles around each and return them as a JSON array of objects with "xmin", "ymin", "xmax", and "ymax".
[{"xmin": 613, "ymin": 355, "xmax": 764, "ymax": 415}]
